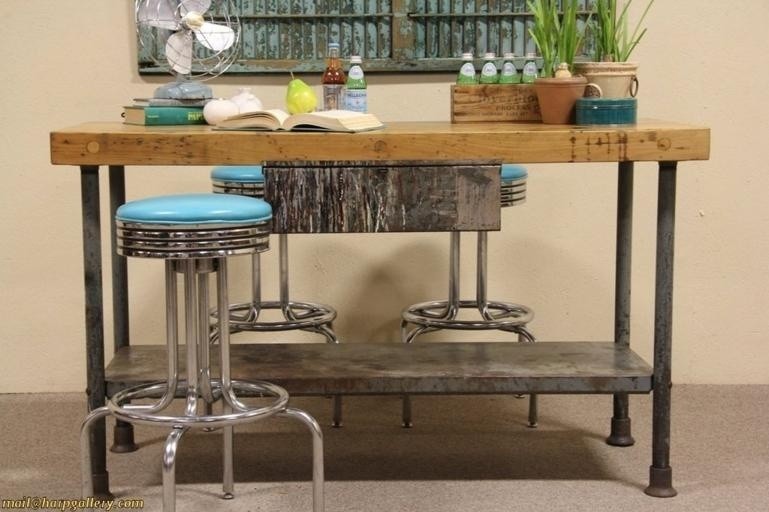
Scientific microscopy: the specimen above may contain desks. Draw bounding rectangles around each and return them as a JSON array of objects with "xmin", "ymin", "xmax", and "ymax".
[{"xmin": 51, "ymin": 115, "xmax": 711, "ymax": 502}]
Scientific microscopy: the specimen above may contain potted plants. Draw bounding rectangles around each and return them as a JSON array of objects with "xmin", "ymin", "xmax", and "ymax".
[{"xmin": 526, "ymin": 0, "xmax": 655, "ymax": 124}]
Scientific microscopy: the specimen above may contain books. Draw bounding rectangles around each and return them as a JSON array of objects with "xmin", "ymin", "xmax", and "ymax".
[
  {"xmin": 211, "ymin": 109, "xmax": 383, "ymax": 134},
  {"xmin": 123, "ymin": 98, "xmax": 214, "ymax": 126}
]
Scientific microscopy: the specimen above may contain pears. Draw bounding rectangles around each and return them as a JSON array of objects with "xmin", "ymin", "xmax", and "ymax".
[{"xmin": 285, "ymin": 70, "xmax": 316, "ymax": 115}]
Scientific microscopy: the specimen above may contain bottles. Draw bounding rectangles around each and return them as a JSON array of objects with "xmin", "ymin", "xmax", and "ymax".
[
  {"xmin": 457, "ymin": 51, "xmax": 539, "ymax": 84},
  {"xmin": 323, "ymin": 43, "xmax": 367, "ymax": 111}
]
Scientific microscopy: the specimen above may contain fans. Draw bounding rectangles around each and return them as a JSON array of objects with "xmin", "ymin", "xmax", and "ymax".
[{"xmin": 134, "ymin": 1, "xmax": 241, "ymax": 101}]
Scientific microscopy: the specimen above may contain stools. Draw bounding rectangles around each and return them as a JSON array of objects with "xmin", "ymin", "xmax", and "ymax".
[{"xmin": 74, "ymin": 164, "xmax": 538, "ymax": 511}]
